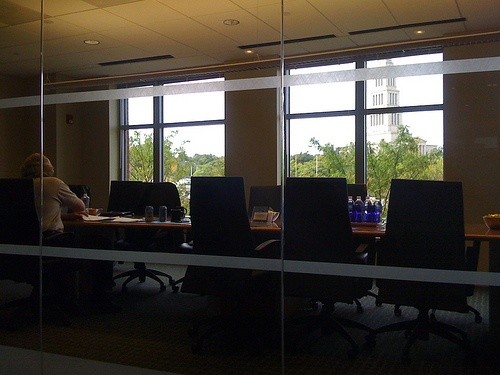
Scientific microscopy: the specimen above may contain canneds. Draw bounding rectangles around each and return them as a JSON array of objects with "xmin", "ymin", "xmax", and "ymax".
[
  {"xmin": 159, "ymin": 206, "xmax": 167, "ymax": 222},
  {"xmin": 145, "ymin": 206, "xmax": 153, "ymax": 222}
]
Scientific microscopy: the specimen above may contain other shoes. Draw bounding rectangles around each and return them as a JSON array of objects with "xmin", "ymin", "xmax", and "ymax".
[{"xmin": 86, "ymin": 292, "xmax": 122, "ymax": 313}]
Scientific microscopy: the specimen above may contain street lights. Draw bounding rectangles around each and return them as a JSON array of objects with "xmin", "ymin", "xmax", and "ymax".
[{"xmin": 184, "ymin": 161, "xmax": 192, "ymax": 176}]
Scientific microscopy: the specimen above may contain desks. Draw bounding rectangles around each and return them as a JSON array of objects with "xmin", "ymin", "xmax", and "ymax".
[{"xmin": 58, "ymin": 209, "xmax": 500, "ymax": 327}]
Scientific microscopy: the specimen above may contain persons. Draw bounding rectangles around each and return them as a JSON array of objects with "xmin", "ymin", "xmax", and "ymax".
[{"xmin": 20, "ymin": 153, "xmax": 121, "ymax": 314}]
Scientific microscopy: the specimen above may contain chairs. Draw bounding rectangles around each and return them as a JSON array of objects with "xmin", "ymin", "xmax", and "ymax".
[{"xmin": 0, "ymin": 176, "xmax": 481, "ymax": 365}]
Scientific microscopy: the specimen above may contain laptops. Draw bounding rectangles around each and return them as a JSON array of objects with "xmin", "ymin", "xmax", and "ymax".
[{"xmin": 101, "ymin": 211, "xmax": 133, "ymax": 216}]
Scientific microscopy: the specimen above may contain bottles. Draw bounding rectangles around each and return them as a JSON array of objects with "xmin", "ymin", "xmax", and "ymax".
[
  {"xmin": 373, "ymin": 198, "xmax": 382, "ymax": 222},
  {"xmin": 348, "ymin": 196, "xmax": 354, "ymax": 222},
  {"xmin": 81, "ymin": 192, "xmax": 89, "ymax": 208},
  {"xmin": 363, "ymin": 197, "xmax": 373, "ymax": 221},
  {"xmin": 267, "ymin": 208, "xmax": 273, "ymax": 226},
  {"xmin": 354, "ymin": 196, "xmax": 364, "ymax": 222}
]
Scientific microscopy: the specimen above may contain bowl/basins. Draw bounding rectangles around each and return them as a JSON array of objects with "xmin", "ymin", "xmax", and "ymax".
[
  {"xmin": 483, "ymin": 216, "xmax": 500, "ymax": 231},
  {"xmin": 86, "ymin": 208, "xmax": 98, "ymax": 215},
  {"xmin": 271, "ymin": 212, "xmax": 280, "ymax": 221}
]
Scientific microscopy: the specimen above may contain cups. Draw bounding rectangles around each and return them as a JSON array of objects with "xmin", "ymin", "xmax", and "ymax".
[
  {"xmin": 170, "ymin": 209, "xmax": 184, "ymax": 223},
  {"xmin": 175, "ymin": 206, "xmax": 187, "ymax": 218},
  {"xmin": 247, "ymin": 207, "xmax": 256, "ymax": 226},
  {"xmin": 376, "ymin": 218, "xmax": 386, "ymax": 231}
]
